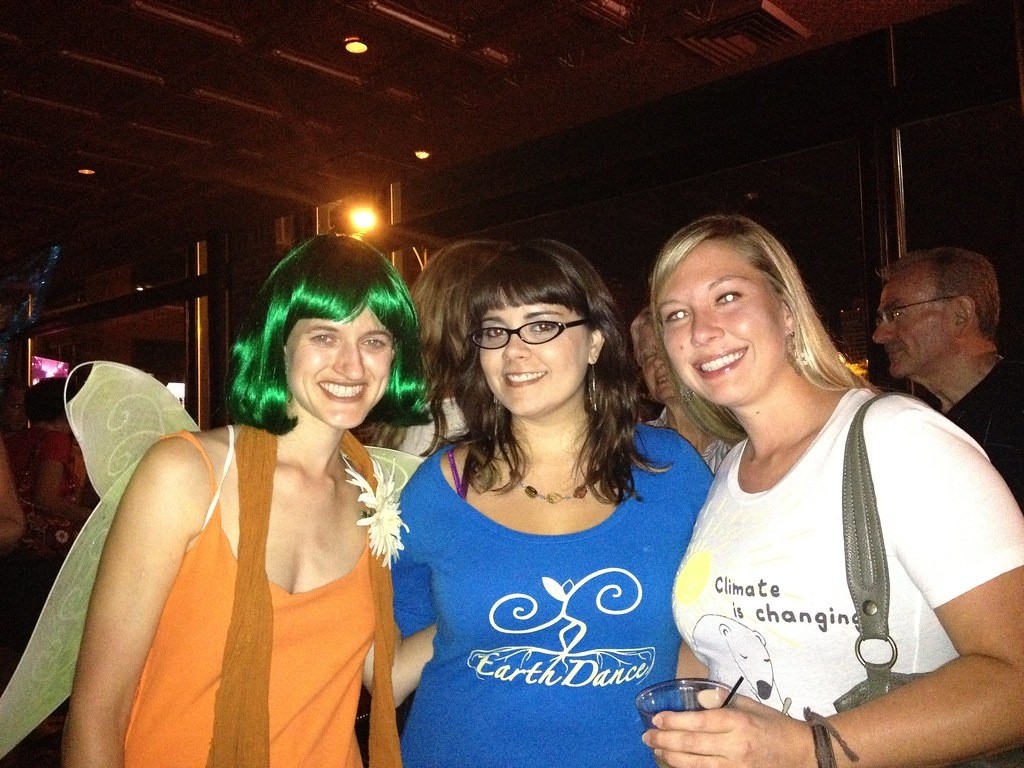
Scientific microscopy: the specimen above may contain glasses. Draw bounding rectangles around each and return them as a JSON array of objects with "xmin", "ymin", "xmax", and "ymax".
[
  {"xmin": 875, "ymin": 295, "xmax": 956, "ymax": 327},
  {"xmin": 466, "ymin": 318, "xmax": 590, "ymax": 349}
]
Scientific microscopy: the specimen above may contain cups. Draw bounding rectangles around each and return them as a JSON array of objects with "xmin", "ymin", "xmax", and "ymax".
[{"xmin": 634, "ymin": 677, "xmax": 737, "ymax": 768}]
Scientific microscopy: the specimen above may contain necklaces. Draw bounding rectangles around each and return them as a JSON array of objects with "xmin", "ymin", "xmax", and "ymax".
[{"xmin": 509, "ymin": 467, "xmax": 592, "ymax": 502}]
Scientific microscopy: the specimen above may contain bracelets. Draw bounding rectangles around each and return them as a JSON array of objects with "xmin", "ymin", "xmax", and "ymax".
[{"xmin": 802, "ymin": 705, "xmax": 860, "ymax": 768}]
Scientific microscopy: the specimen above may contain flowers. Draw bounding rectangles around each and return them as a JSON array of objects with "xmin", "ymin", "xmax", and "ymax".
[{"xmin": 343, "ymin": 455, "xmax": 411, "ymax": 569}]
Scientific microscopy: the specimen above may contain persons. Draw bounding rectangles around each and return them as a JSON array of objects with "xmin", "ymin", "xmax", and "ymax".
[
  {"xmin": 357, "ymin": 241, "xmax": 501, "ymax": 459},
  {"xmin": 628, "ymin": 306, "xmax": 732, "ymax": 479},
  {"xmin": 0, "ymin": 376, "xmax": 100, "ymax": 738},
  {"xmin": 390, "ymin": 239, "xmax": 715, "ymax": 768},
  {"xmin": 641, "ymin": 215, "xmax": 1024, "ymax": 768},
  {"xmin": 61, "ymin": 235, "xmax": 436, "ymax": 767},
  {"xmin": 870, "ymin": 250, "xmax": 1023, "ymax": 513}
]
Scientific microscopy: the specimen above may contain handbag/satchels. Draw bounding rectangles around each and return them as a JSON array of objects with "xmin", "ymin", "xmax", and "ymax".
[
  {"xmin": 9, "ymin": 480, "xmax": 92, "ymax": 560},
  {"xmin": 833, "ymin": 671, "xmax": 925, "ymax": 712}
]
